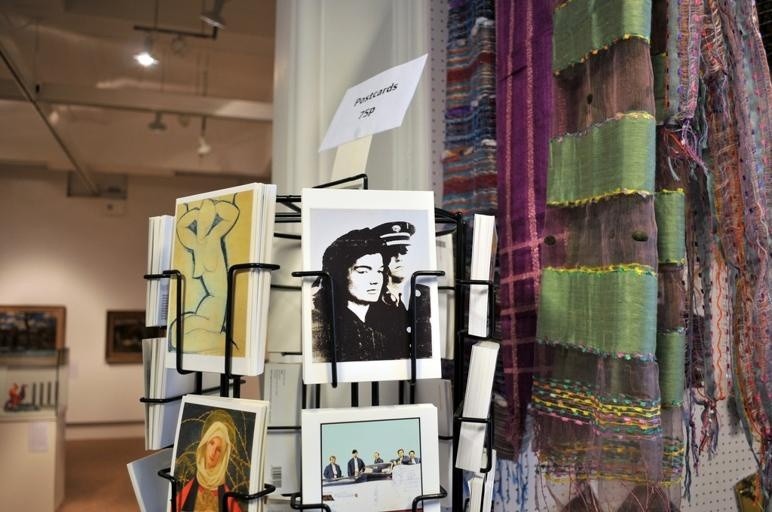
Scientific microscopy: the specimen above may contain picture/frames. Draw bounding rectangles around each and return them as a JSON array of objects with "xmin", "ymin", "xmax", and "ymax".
[
  {"xmin": 0, "ymin": 305, "xmax": 66, "ymax": 368},
  {"xmin": 106, "ymin": 310, "xmax": 145, "ymax": 364}
]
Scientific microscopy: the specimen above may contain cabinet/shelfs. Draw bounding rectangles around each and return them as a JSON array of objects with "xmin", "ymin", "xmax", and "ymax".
[{"xmin": 135, "ymin": 171, "xmax": 501, "ymax": 511}]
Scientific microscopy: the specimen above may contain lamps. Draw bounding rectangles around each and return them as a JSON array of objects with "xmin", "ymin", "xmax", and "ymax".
[{"xmin": 134, "ymin": 0, "xmax": 229, "ymax": 154}]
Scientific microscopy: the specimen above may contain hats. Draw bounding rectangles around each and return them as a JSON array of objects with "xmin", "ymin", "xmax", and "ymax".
[{"xmin": 372, "ymin": 221, "xmax": 414, "ymax": 246}]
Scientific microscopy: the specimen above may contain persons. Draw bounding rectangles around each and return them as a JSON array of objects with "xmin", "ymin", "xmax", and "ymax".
[
  {"xmin": 315, "ymin": 227, "xmax": 405, "ymax": 359},
  {"xmin": 371, "ymin": 220, "xmax": 432, "ymax": 362},
  {"xmin": 322, "ymin": 444, "xmax": 421, "ymax": 483},
  {"xmin": 169, "ymin": 421, "xmax": 249, "ymax": 511}
]
